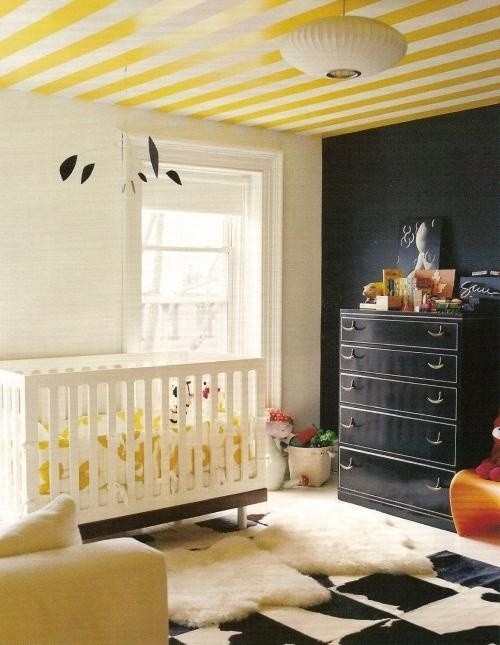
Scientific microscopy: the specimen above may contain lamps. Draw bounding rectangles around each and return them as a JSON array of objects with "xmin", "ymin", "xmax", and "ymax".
[{"xmin": 279, "ymin": 12, "xmax": 409, "ymax": 87}]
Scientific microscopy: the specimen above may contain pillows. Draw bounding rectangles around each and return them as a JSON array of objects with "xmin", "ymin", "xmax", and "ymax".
[{"xmin": 0, "ymin": 493, "xmax": 79, "ymax": 554}]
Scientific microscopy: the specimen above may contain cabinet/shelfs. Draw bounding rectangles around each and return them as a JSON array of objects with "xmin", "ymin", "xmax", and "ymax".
[{"xmin": 337, "ymin": 311, "xmax": 499, "ymax": 526}]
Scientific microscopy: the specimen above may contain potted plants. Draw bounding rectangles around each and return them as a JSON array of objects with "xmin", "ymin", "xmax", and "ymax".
[{"xmin": 287, "ymin": 429, "xmax": 337, "ymax": 487}]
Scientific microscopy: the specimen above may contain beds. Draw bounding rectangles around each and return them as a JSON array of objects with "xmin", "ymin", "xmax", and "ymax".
[{"xmin": 0, "ymin": 351, "xmax": 267, "ymax": 538}]
